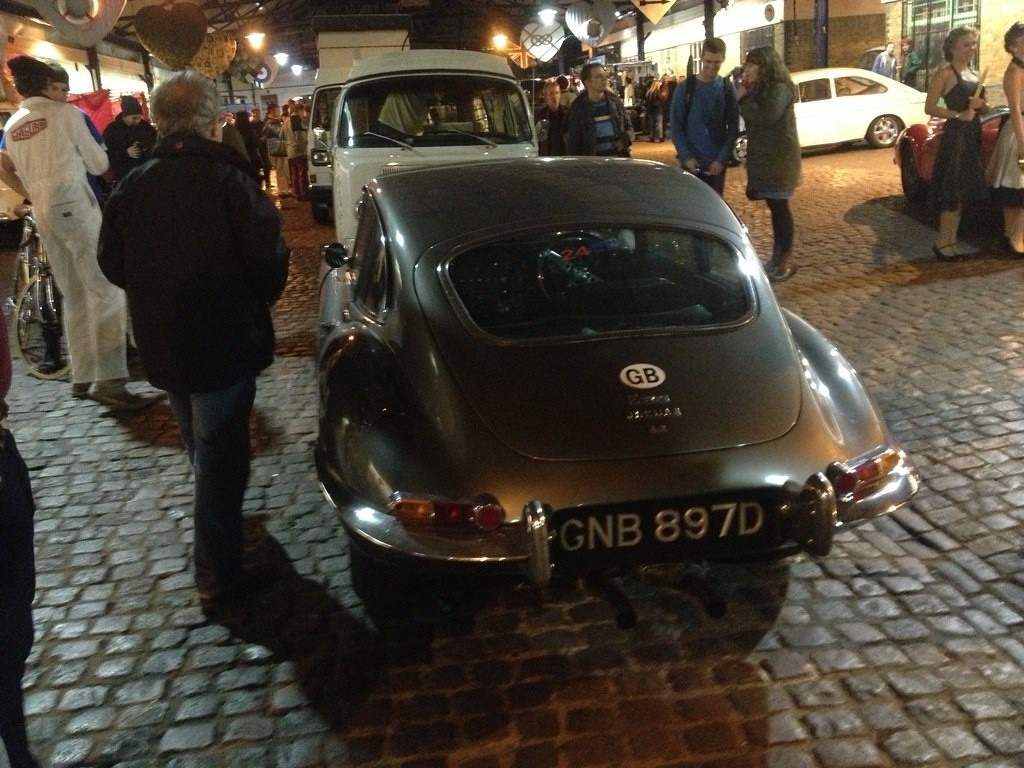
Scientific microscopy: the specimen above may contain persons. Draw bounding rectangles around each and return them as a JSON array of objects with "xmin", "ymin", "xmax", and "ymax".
[
  {"xmin": 900, "ymin": 38, "xmax": 923, "ymax": 87},
  {"xmin": 622, "ymin": 74, "xmax": 688, "ymax": 142},
  {"xmin": 214, "ymin": 98, "xmax": 314, "ymax": 194},
  {"xmin": 96, "ymin": 71, "xmax": 296, "ymax": 626},
  {"xmin": 987, "ymin": 22, "xmax": 1024, "ymax": 260},
  {"xmin": 0, "ymin": 49, "xmax": 165, "ymax": 415},
  {"xmin": 534, "ymin": 75, "xmax": 578, "ymax": 158},
  {"xmin": 872, "ymin": 42, "xmax": 897, "ymax": 80},
  {"xmin": 567, "ymin": 64, "xmax": 635, "ymax": 158},
  {"xmin": 924, "ymin": 24, "xmax": 991, "ymax": 262},
  {"xmin": 0, "ymin": 302, "xmax": 41, "ymax": 768},
  {"xmin": 735, "ymin": 47, "xmax": 805, "ymax": 282},
  {"xmin": 669, "ymin": 37, "xmax": 739, "ymax": 199}
]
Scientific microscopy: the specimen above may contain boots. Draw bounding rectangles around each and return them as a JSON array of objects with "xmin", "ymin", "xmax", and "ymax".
[{"xmin": 37, "ymin": 322, "xmax": 63, "ymax": 371}]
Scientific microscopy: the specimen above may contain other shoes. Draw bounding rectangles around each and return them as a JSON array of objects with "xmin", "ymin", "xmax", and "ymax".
[
  {"xmin": 71, "ymin": 378, "xmax": 152, "ymax": 407},
  {"xmin": 764, "ymin": 251, "xmax": 797, "ymax": 281},
  {"xmin": 932, "ymin": 242, "xmax": 969, "ymax": 261}
]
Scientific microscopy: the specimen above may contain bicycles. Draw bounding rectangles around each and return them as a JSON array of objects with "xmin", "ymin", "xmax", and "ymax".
[{"xmin": 11, "ymin": 210, "xmax": 72, "ymax": 380}]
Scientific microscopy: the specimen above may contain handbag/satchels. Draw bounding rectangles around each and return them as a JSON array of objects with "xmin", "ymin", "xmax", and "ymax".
[{"xmin": 267, "ymin": 137, "xmax": 287, "ymax": 158}]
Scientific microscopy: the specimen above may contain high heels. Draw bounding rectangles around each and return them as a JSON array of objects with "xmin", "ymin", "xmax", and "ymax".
[{"xmin": 1001, "ymin": 233, "xmax": 1024, "ymax": 258}]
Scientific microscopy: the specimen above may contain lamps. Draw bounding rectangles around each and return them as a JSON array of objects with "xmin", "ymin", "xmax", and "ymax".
[
  {"xmin": 245, "ymin": 23, "xmax": 265, "ymax": 49},
  {"xmin": 538, "ymin": 0, "xmax": 558, "ymax": 26}
]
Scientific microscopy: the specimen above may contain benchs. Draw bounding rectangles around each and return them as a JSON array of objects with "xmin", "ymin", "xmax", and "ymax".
[{"xmin": 466, "ymin": 278, "xmax": 719, "ymax": 337}]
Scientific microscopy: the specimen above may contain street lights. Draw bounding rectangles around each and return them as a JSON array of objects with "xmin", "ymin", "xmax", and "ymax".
[
  {"xmin": 228, "ymin": 30, "xmax": 301, "ymax": 108},
  {"xmin": 494, "ymin": 35, "xmax": 536, "ymax": 118}
]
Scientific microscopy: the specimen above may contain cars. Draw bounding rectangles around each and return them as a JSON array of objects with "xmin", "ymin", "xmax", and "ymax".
[
  {"xmin": 857, "ymin": 45, "xmax": 885, "ymax": 71},
  {"xmin": 306, "ymin": 48, "xmax": 549, "ymax": 265},
  {"xmin": 730, "ymin": 65, "xmax": 929, "ymax": 164},
  {"xmin": 0, "ymin": 98, "xmax": 32, "ymax": 241},
  {"xmin": 895, "ymin": 107, "xmax": 1009, "ymax": 207},
  {"xmin": 313, "ymin": 154, "xmax": 923, "ymax": 633}
]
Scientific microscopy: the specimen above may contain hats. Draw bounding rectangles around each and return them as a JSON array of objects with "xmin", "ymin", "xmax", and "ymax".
[
  {"xmin": 121, "ymin": 95, "xmax": 142, "ymax": 116},
  {"xmin": 7, "ymin": 56, "xmax": 56, "ymax": 79},
  {"xmin": 48, "ymin": 63, "xmax": 71, "ymax": 84}
]
